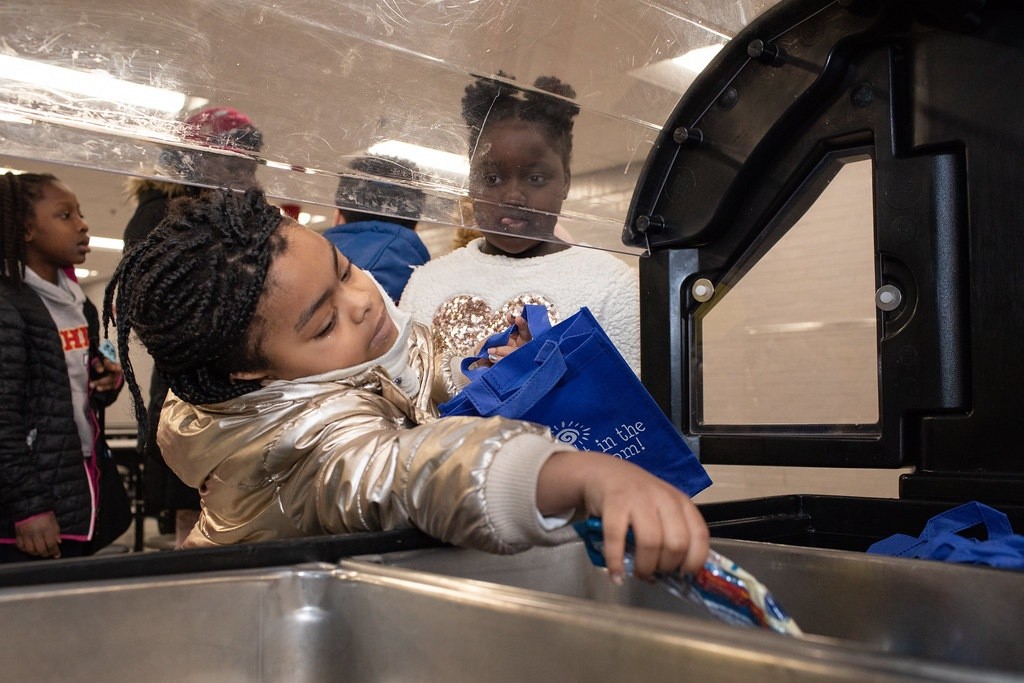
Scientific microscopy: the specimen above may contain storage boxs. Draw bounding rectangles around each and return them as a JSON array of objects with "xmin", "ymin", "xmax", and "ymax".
[
  {"xmin": 340, "ymin": 535, "xmax": 1023, "ymax": 682},
  {"xmin": 0, "ymin": 560, "xmax": 919, "ymax": 683}
]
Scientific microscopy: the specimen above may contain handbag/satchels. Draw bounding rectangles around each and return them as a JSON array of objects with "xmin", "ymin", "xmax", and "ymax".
[
  {"xmin": 869, "ymin": 504, "xmax": 1023, "ymax": 575},
  {"xmin": 17, "ymin": 455, "xmax": 134, "ymax": 558},
  {"xmin": 440, "ymin": 314, "xmax": 710, "ymax": 567}
]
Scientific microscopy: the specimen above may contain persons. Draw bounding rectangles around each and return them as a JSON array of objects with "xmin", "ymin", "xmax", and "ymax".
[
  {"xmin": 316, "ymin": 151, "xmax": 433, "ymax": 312},
  {"xmin": 123, "ymin": 107, "xmax": 267, "ymax": 539},
  {"xmin": 397, "ymin": 68, "xmax": 644, "ymax": 415},
  {"xmin": 1, "ymin": 168, "xmax": 131, "ymax": 573},
  {"xmin": 100, "ymin": 183, "xmax": 712, "ymax": 590}
]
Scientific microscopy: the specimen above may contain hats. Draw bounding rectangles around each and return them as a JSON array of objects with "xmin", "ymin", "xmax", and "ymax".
[{"xmin": 182, "ymin": 109, "xmax": 262, "ymax": 156}]
